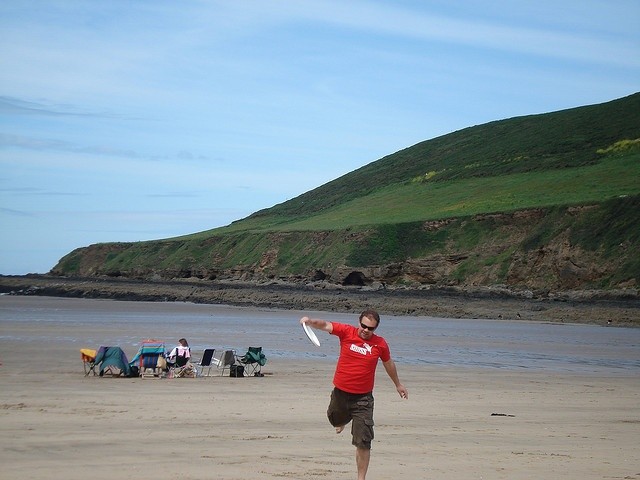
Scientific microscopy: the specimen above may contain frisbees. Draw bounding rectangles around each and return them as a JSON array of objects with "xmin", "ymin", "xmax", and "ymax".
[{"xmin": 302, "ymin": 322, "xmax": 320, "ymax": 348}]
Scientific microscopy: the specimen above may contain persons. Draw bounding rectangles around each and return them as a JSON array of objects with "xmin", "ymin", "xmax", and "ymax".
[
  {"xmin": 166, "ymin": 338, "xmax": 189, "ymax": 363},
  {"xmin": 301, "ymin": 310, "xmax": 408, "ymax": 479}
]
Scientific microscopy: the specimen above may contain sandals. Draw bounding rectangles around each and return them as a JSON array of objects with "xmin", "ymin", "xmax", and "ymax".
[{"xmin": 254, "ymin": 371, "xmax": 264, "ymax": 377}]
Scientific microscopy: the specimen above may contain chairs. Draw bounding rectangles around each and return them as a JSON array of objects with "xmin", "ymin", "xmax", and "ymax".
[
  {"xmin": 212, "ymin": 349, "xmax": 238, "ymax": 377},
  {"xmin": 189, "ymin": 348, "xmax": 216, "ymax": 377},
  {"xmin": 94, "ymin": 345, "xmax": 131, "ymax": 378},
  {"xmin": 80, "ymin": 348, "xmax": 113, "ymax": 376},
  {"xmin": 166, "ymin": 348, "xmax": 191, "ymax": 378},
  {"xmin": 129, "ymin": 338, "xmax": 167, "ymax": 380},
  {"xmin": 236, "ymin": 346, "xmax": 267, "ymax": 377}
]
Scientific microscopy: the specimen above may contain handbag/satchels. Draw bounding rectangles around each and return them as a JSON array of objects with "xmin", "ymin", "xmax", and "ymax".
[
  {"xmin": 231, "ymin": 365, "xmax": 244, "ymax": 377},
  {"xmin": 156, "ymin": 355, "xmax": 167, "ymax": 370}
]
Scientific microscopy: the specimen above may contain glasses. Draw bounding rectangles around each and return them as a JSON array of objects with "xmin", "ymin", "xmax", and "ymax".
[{"xmin": 360, "ymin": 323, "xmax": 375, "ymax": 331}]
